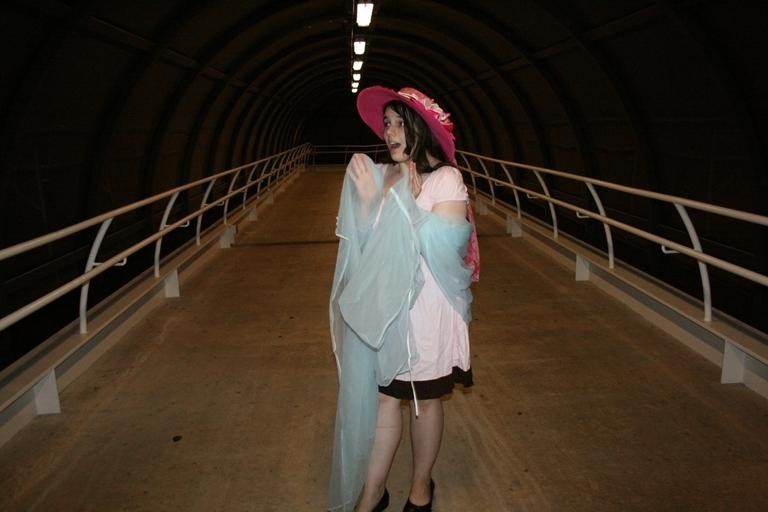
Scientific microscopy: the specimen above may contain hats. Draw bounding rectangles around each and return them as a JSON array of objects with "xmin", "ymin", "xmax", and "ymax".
[{"xmin": 356, "ymin": 86, "xmax": 456, "ymax": 162}]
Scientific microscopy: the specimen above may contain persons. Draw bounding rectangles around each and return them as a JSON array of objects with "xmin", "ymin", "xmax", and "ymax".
[{"xmin": 345, "ymin": 82, "xmax": 478, "ymax": 512}]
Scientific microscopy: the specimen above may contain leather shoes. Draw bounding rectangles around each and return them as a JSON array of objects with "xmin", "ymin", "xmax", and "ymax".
[
  {"xmin": 372, "ymin": 489, "xmax": 390, "ymax": 512},
  {"xmin": 403, "ymin": 478, "xmax": 434, "ymax": 510}
]
